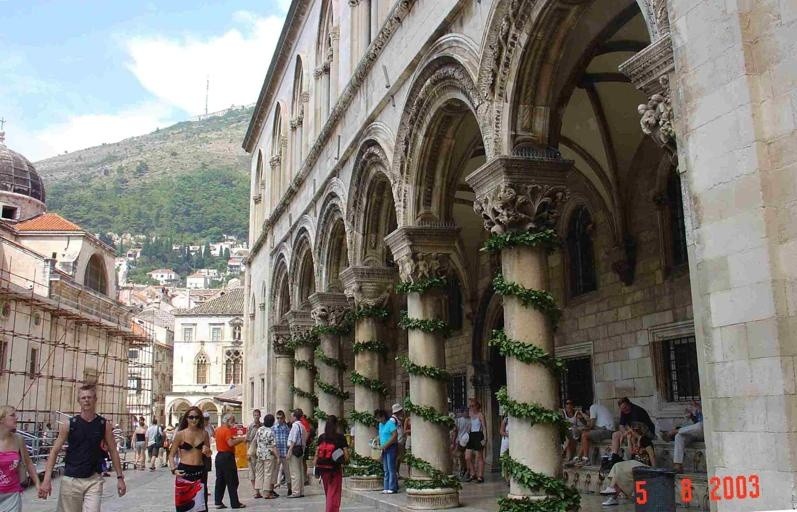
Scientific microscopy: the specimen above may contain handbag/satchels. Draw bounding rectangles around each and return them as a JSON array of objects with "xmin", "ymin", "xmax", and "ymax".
[
  {"xmin": 155, "ymin": 434, "xmax": 169, "ymax": 448},
  {"xmin": 372, "ymin": 448, "xmax": 383, "ymax": 461},
  {"xmin": 460, "ymin": 432, "xmax": 470, "ymax": 447},
  {"xmin": 292, "ymin": 445, "xmax": 303, "ymax": 457},
  {"xmin": 19, "ymin": 463, "xmax": 29, "ymax": 486},
  {"xmin": 573, "ymin": 427, "xmax": 580, "ymax": 441},
  {"xmin": 247, "ymin": 438, "xmax": 256, "ymax": 456}
]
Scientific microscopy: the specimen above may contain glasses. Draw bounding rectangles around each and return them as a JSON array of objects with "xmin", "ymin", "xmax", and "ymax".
[{"xmin": 188, "ymin": 416, "xmax": 200, "ymax": 419}]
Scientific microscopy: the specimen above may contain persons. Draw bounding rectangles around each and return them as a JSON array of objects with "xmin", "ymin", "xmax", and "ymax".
[
  {"xmin": 167, "ymin": 405, "xmax": 212, "ymax": 512},
  {"xmin": 209, "ymin": 413, "xmax": 248, "ymax": 508},
  {"xmin": 370, "ymin": 395, "xmax": 508, "ymax": 494},
  {"xmin": 655, "ymin": 398, "xmax": 703, "ymax": 475},
  {"xmin": 560, "ymin": 396, "xmax": 655, "ymax": 506},
  {"xmin": 43, "ymin": 422, "xmax": 55, "ymax": 459},
  {"xmin": 313, "ymin": 412, "xmax": 350, "ymax": 511},
  {"xmin": 38, "ymin": 384, "xmax": 127, "ymax": 511},
  {"xmin": 0, "ymin": 404, "xmax": 43, "ymax": 511},
  {"xmin": 99, "ymin": 407, "xmax": 311, "ymax": 498}
]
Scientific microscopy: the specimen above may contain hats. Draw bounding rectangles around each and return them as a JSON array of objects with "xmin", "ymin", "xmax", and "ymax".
[{"xmin": 392, "ymin": 403, "xmax": 403, "ymax": 413}]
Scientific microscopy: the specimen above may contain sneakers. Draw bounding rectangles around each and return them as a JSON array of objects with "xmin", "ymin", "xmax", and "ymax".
[
  {"xmin": 670, "ymin": 469, "xmax": 683, "ymax": 474},
  {"xmin": 215, "ymin": 503, "xmax": 246, "ymax": 509},
  {"xmin": 287, "ymin": 489, "xmax": 304, "ymax": 498},
  {"xmin": 381, "ymin": 490, "xmax": 395, "ymax": 494},
  {"xmin": 150, "ymin": 463, "xmax": 168, "ymax": 470},
  {"xmin": 600, "ymin": 487, "xmax": 616, "ymax": 496},
  {"xmin": 602, "ymin": 497, "xmax": 618, "ymax": 505},
  {"xmin": 667, "ymin": 430, "xmax": 676, "ymax": 437},
  {"xmin": 565, "ymin": 457, "xmax": 591, "ymax": 466},
  {"xmin": 459, "ymin": 472, "xmax": 484, "ymax": 482}
]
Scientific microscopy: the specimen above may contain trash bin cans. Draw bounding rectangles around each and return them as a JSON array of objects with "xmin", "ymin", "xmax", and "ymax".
[{"xmin": 632, "ymin": 465, "xmax": 676, "ymax": 512}]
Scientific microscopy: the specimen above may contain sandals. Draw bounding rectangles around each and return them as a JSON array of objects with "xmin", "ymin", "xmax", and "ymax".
[{"xmin": 254, "ymin": 490, "xmax": 279, "ymax": 498}]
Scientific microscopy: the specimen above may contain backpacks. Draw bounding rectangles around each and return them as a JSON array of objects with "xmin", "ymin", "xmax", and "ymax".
[{"xmin": 317, "ymin": 440, "xmax": 338, "ymax": 472}]
